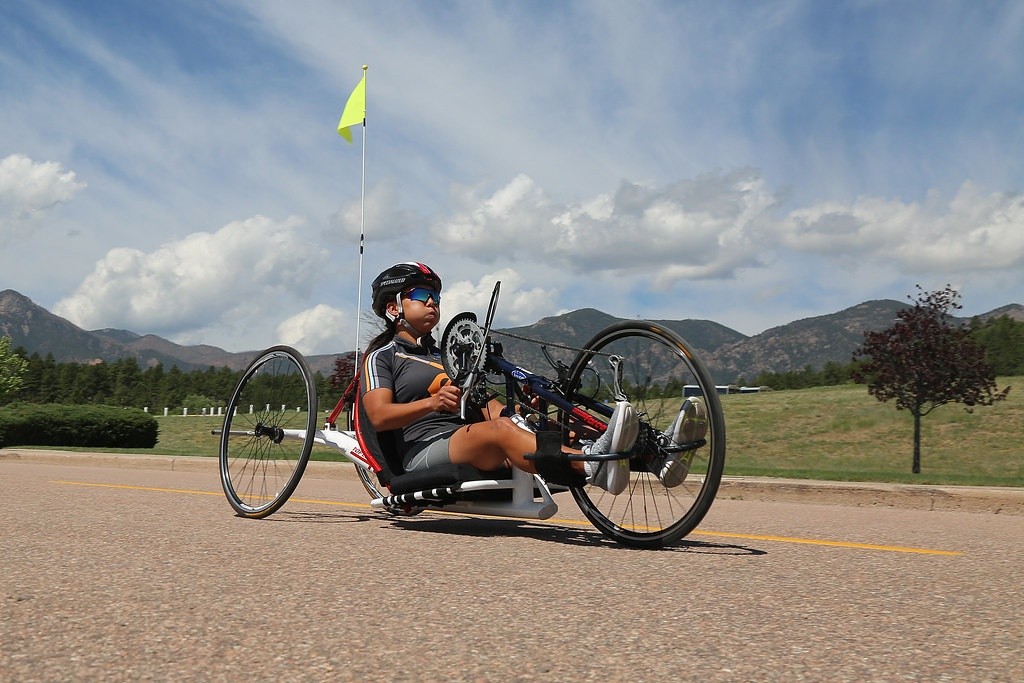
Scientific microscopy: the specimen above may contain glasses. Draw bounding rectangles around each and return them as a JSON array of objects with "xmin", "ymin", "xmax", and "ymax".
[{"xmin": 402, "ymin": 287, "xmax": 439, "ymax": 303}]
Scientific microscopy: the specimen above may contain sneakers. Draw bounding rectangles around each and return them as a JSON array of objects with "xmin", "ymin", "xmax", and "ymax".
[
  {"xmin": 582, "ymin": 402, "xmax": 639, "ymax": 495},
  {"xmin": 647, "ymin": 396, "xmax": 708, "ymax": 487}
]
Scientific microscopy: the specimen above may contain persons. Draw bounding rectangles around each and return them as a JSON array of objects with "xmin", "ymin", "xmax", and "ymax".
[{"xmin": 361, "ymin": 261, "xmax": 707, "ymax": 496}]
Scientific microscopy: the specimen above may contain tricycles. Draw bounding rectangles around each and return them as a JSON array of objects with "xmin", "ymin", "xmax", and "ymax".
[{"xmin": 210, "ymin": 280, "xmax": 726, "ymax": 550}]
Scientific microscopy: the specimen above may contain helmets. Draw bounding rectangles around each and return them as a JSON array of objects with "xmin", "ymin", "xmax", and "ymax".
[{"xmin": 371, "ymin": 262, "xmax": 441, "ymax": 319}]
